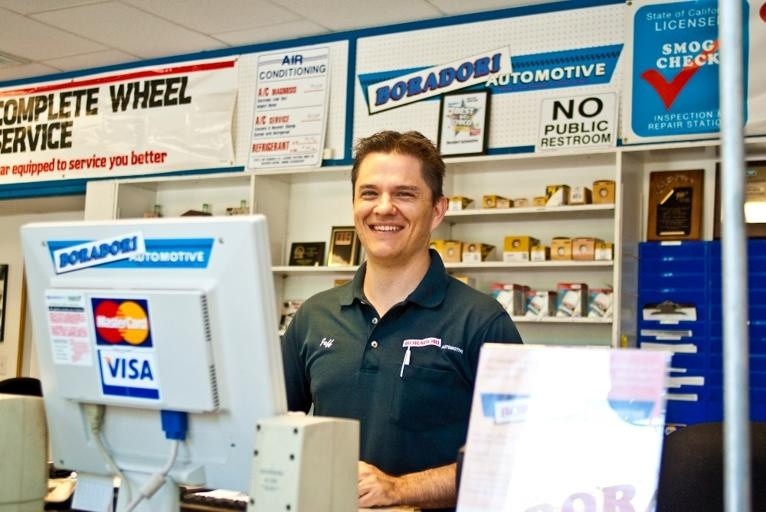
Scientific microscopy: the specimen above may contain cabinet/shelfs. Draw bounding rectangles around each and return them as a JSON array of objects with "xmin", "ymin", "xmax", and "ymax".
[{"xmin": 112, "ymin": 147, "xmax": 619, "ymax": 347}]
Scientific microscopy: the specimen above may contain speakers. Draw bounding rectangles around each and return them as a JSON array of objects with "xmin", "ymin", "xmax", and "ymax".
[{"xmin": 248, "ymin": 415, "xmax": 361, "ymax": 512}]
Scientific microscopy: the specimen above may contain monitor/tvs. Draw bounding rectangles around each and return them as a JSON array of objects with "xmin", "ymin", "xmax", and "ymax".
[{"xmin": 19, "ymin": 213, "xmax": 287, "ymax": 512}]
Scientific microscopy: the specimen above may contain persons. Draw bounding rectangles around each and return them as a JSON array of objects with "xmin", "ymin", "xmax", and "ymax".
[{"xmin": 281, "ymin": 129, "xmax": 524, "ymax": 509}]
